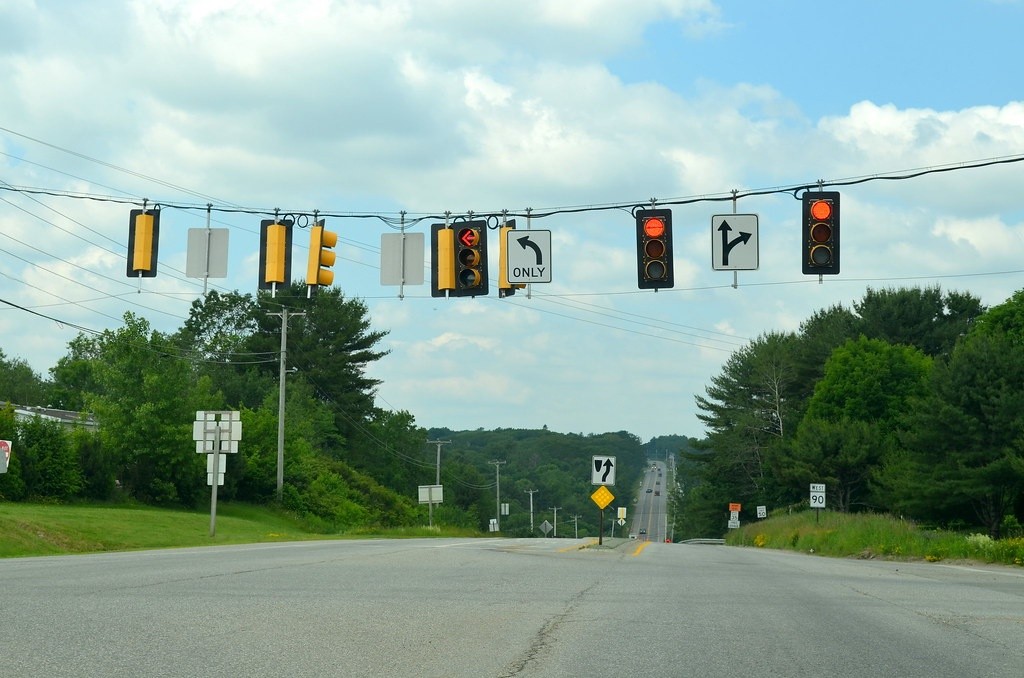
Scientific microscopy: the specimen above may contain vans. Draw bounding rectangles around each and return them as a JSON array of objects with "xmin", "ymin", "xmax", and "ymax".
[{"xmin": 629, "ymin": 534, "xmax": 638, "ymax": 539}]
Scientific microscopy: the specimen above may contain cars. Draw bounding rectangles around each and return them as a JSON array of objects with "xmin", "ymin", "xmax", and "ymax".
[
  {"xmin": 646, "ymin": 488, "xmax": 652, "ymax": 493},
  {"xmin": 639, "ymin": 528, "xmax": 647, "ymax": 534},
  {"xmin": 657, "ymin": 472, "xmax": 662, "ymax": 477},
  {"xmin": 656, "ymin": 481, "xmax": 661, "ymax": 485},
  {"xmin": 657, "ymin": 467, "xmax": 661, "ymax": 470},
  {"xmin": 654, "ymin": 490, "xmax": 660, "ymax": 496}
]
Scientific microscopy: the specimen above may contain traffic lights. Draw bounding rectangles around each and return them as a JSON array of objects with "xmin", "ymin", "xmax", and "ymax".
[
  {"xmin": 452, "ymin": 220, "xmax": 489, "ymax": 298},
  {"xmin": 803, "ymin": 189, "xmax": 841, "ymax": 278},
  {"xmin": 307, "ymin": 220, "xmax": 337, "ymax": 292},
  {"xmin": 636, "ymin": 207, "xmax": 673, "ymax": 289}
]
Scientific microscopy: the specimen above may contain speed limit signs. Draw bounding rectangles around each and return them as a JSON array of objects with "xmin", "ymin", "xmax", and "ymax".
[
  {"xmin": 809, "ymin": 482, "xmax": 826, "ymax": 526},
  {"xmin": 756, "ymin": 506, "xmax": 767, "ymax": 522}
]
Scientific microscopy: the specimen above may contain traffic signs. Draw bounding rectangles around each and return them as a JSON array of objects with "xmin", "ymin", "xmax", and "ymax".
[
  {"xmin": 506, "ymin": 230, "xmax": 552, "ymax": 285},
  {"xmin": 711, "ymin": 212, "xmax": 760, "ymax": 272}
]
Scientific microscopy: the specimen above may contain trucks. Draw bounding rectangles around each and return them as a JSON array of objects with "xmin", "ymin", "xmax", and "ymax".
[{"xmin": 651, "ymin": 461, "xmax": 657, "ymax": 470}]
